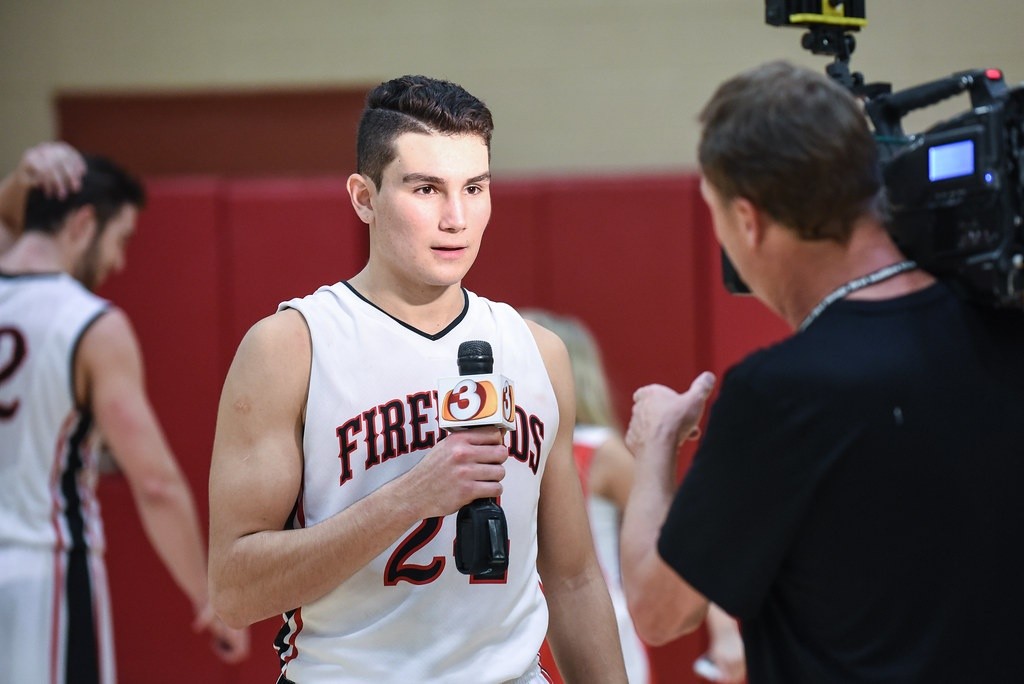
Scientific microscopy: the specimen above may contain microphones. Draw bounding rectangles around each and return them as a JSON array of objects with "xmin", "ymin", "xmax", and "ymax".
[{"xmin": 436, "ymin": 340, "xmax": 515, "ymax": 579}]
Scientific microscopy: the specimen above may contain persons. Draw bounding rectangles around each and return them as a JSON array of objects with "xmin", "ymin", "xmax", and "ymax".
[
  {"xmin": 0, "ymin": 141, "xmax": 247, "ymax": 684},
  {"xmin": 617, "ymin": 57, "xmax": 1024, "ymax": 684},
  {"xmin": 208, "ymin": 75, "xmax": 628, "ymax": 684},
  {"xmin": 512, "ymin": 311, "xmax": 746, "ymax": 684}
]
